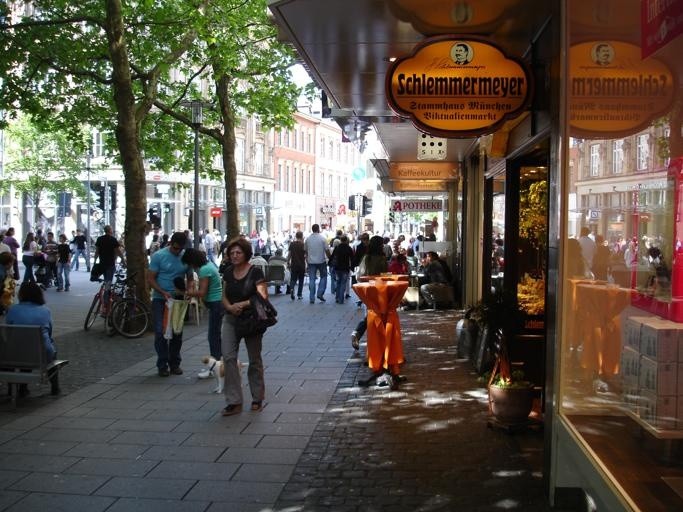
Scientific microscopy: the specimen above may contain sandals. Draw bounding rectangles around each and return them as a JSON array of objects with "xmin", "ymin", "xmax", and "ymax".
[
  {"xmin": 252, "ymin": 400, "xmax": 262, "ymax": 409},
  {"xmin": 221, "ymin": 405, "xmax": 242, "ymax": 415}
]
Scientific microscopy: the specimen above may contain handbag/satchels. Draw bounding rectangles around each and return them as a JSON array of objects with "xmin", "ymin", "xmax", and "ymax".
[{"xmin": 162, "ymin": 296, "xmax": 189, "ymax": 340}]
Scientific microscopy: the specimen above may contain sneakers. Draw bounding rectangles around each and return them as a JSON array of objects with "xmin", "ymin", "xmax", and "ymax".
[
  {"xmin": 198, "ymin": 369, "xmax": 216, "ymax": 379},
  {"xmin": 351, "ymin": 331, "xmax": 359, "ymax": 349}
]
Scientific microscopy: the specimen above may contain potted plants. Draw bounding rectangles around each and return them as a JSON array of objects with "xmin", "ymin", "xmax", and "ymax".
[{"xmin": 464, "ymin": 290, "xmax": 535, "ymax": 423}]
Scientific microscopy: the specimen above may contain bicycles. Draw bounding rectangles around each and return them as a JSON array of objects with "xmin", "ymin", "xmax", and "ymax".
[{"xmin": 84, "ymin": 267, "xmax": 150, "ymax": 338}]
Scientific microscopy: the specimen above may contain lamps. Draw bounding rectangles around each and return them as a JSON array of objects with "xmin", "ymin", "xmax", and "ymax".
[{"xmin": 417, "ymin": 130, "xmax": 447, "ymax": 160}]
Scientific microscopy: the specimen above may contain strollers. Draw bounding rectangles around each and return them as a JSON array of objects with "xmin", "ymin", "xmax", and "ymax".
[{"xmin": 35, "ymin": 250, "xmax": 59, "ymax": 289}]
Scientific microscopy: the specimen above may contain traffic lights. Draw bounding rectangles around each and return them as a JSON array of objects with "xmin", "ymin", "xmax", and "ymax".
[
  {"xmin": 147, "ymin": 206, "xmax": 161, "ymax": 229},
  {"xmin": 164, "ymin": 203, "xmax": 170, "ymax": 213},
  {"xmin": 347, "ymin": 194, "xmax": 355, "ymax": 210},
  {"xmin": 108, "ymin": 185, "xmax": 118, "ymax": 211},
  {"xmin": 388, "ymin": 207, "xmax": 394, "ymax": 223},
  {"xmin": 361, "ymin": 196, "xmax": 372, "ymax": 216},
  {"xmin": 95, "ymin": 189, "xmax": 104, "ymax": 211}
]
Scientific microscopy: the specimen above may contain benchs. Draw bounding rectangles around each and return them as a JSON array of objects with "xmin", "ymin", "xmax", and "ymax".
[
  {"xmin": 255, "ymin": 265, "xmax": 284, "ymax": 294},
  {"xmin": 0, "ymin": 325, "xmax": 69, "ymax": 413}
]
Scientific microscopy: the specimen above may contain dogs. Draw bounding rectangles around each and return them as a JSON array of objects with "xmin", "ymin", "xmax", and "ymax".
[{"xmin": 200, "ymin": 354, "xmax": 249, "ymax": 394}]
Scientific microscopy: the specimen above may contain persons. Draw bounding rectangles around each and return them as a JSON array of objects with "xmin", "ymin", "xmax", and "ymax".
[
  {"xmin": 180, "ymin": 248, "xmax": 224, "ymax": 378},
  {"xmin": 595, "ymin": 44, "xmax": 611, "ymax": 65},
  {"xmin": 330, "ymin": 230, "xmax": 372, "ymax": 307},
  {"xmin": 6, "ymin": 282, "xmax": 62, "ymax": 397},
  {"xmin": 148, "ymin": 231, "xmax": 194, "ymax": 377},
  {"xmin": 287, "ymin": 231, "xmax": 306, "ymax": 301},
  {"xmin": 385, "ymin": 234, "xmax": 452, "ymax": 308},
  {"xmin": 204, "ymin": 228, "xmax": 218, "ymax": 269},
  {"xmin": 492, "ymin": 238, "xmax": 504, "ymax": 259},
  {"xmin": 150, "ymin": 226, "xmax": 192, "ymax": 255},
  {"xmin": 1, "ymin": 227, "xmax": 19, "ymax": 317},
  {"xmin": 243, "ymin": 228, "xmax": 294, "ymax": 296},
  {"xmin": 304, "ymin": 224, "xmax": 332, "ymax": 304},
  {"xmin": 328, "ymin": 236, "xmax": 353, "ymax": 304},
  {"xmin": 221, "ymin": 237, "xmax": 267, "ymax": 415},
  {"xmin": 94, "ymin": 225, "xmax": 127, "ymax": 289},
  {"xmin": 453, "ymin": 44, "xmax": 471, "ymax": 65},
  {"xmin": 22, "ymin": 228, "xmax": 91, "ymax": 292},
  {"xmin": 351, "ymin": 236, "xmax": 387, "ymax": 361},
  {"xmin": 566, "ymin": 228, "xmax": 673, "ymax": 301}
]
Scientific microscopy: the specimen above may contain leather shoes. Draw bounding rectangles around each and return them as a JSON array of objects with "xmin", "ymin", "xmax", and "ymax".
[{"xmin": 159, "ymin": 366, "xmax": 182, "ymax": 376}]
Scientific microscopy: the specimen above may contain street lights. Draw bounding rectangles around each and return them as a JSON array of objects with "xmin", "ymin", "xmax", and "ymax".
[{"xmin": 180, "ymin": 99, "xmax": 215, "ymax": 250}]
